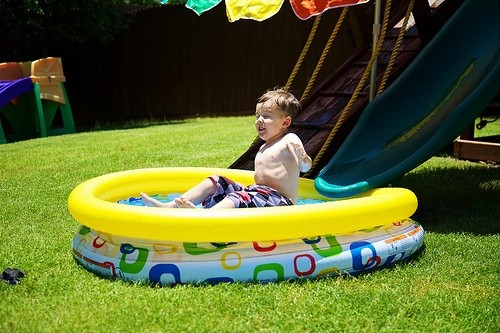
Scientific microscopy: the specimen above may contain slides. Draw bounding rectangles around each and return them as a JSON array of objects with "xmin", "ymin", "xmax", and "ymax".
[{"xmin": 313, "ymin": 0, "xmax": 500, "ymax": 199}]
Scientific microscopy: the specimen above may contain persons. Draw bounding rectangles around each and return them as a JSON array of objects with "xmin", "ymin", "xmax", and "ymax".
[{"xmin": 140, "ymin": 89, "xmax": 312, "ymax": 209}]
